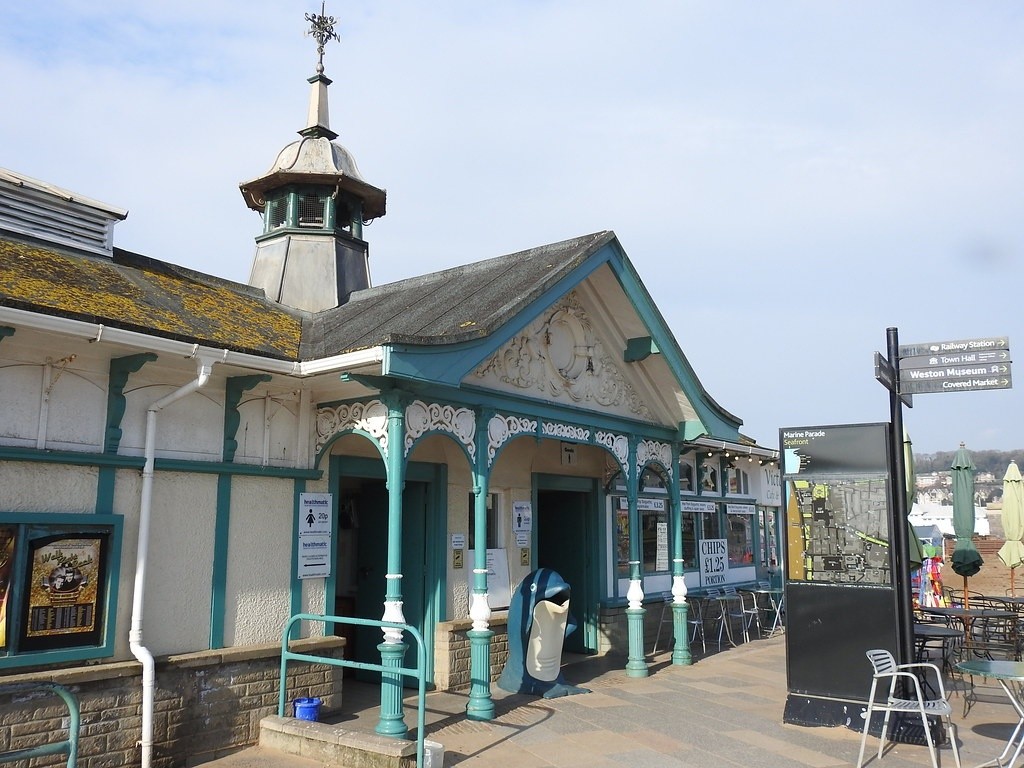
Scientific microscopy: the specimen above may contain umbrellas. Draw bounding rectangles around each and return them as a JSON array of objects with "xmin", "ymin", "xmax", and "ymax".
[
  {"xmin": 903, "ymin": 429, "xmax": 924, "ymax": 574},
  {"xmin": 951, "ymin": 441, "xmax": 985, "ymax": 650},
  {"xmin": 997, "ymin": 457, "xmax": 1024, "ymax": 596}
]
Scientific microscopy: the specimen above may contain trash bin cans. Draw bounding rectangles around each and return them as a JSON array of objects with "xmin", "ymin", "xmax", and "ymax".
[{"xmin": 293, "ymin": 697, "xmax": 321, "ymax": 722}]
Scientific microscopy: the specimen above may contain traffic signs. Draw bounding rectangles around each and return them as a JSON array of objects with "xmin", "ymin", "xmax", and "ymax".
[
  {"xmin": 898, "ymin": 335, "xmax": 1012, "ymax": 395},
  {"xmin": 873, "ymin": 351, "xmax": 895, "ymax": 392}
]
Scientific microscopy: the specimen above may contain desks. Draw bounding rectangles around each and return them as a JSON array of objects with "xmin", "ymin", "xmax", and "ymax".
[
  {"xmin": 956, "ymin": 658, "xmax": 1024, "ymax": 768},
  {"xmin": 753, "ymin": 587, "xmax": 785, "ymax": 638},
  {"xmin": 684, "ymin": 594, "xmax": 739, "ymax": 653}
]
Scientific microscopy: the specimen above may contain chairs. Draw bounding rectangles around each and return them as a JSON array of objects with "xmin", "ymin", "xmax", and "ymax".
[
  {"xmin": 856, "ymin": 647, "xmax": 963, "ymax": 768},
  {"xmin": 759, "ymin": 581, "xmax": 787, "ymax": 630},
  {"xmin": 697, "ymin": 587, "xmax": 734, "ymax": 645},
  {"xmin": 912, "ymin": 585, "xmax": 1024, "ymax": 701},
  {"xmin": 723, "ymin": 585, "xmax": 761, "ymax": 644},
  {"xmin": 653, "ymin": 591, "xmax": 695, "ymax": 655}
]
